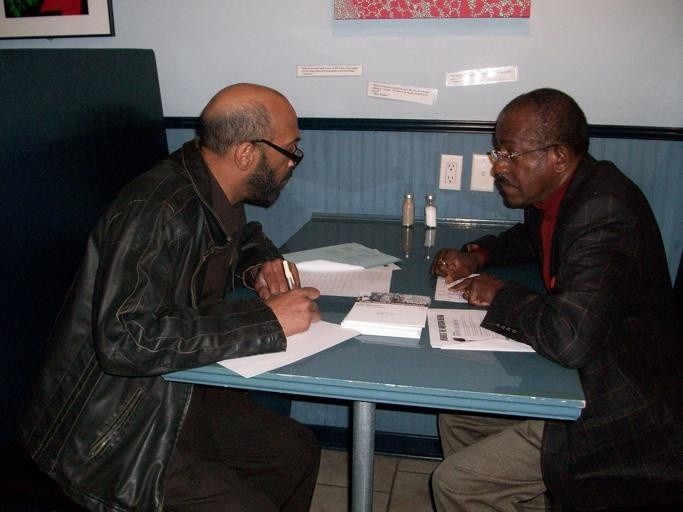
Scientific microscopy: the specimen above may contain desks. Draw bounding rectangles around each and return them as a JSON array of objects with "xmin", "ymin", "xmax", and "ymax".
[{"xmin": 161, "ymin": 214, "xmax": 587, "ymax": 512}]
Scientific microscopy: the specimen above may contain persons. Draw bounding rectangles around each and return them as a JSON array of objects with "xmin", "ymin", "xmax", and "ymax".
[
  {"xmin": 14, "ymin": 82, "xmax": 321, "ymax": 507},
  {"xmin": 427, "ymin": 87, "xmax": 682, "ymax": 512}
]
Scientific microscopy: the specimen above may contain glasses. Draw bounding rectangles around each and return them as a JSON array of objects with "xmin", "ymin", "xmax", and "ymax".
[
  {"xmin": 250, "ymin": 140, "xmax": 304, "ymax": 168},
  {"xmin": 487, "ymin": 145, "xmax": 558, "ymax": 166}
]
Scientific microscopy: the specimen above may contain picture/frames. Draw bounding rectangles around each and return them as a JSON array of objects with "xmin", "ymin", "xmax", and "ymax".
[{"xmin": 0, "ymin": 0, "xmax": 115, "ymax": 40}]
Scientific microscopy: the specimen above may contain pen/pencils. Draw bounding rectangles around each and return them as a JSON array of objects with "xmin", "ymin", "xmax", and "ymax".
[{"xmin": 282, "ymin": 260, "xmax": 295, "ymax": 291}]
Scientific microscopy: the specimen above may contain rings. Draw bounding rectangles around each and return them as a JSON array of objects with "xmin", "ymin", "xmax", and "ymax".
[
  {"xmin": 465, "ymin": 287, "xmax": 471, "ymax": 295},
  {"xmin": 437, "ymin": 258, "xmax": 447, "ymax": 267}
]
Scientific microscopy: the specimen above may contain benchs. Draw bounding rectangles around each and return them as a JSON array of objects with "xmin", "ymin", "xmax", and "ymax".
[{"xmin": 0, "ymin": 48, "xmax": 291, "ymax": 511}]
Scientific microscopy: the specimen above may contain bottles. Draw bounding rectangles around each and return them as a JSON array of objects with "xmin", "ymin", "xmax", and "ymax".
[
  {"xmin": 425, "ymin": 196, "xmax": 437, "ymax": 228},
  {"xmin": 401, "ymin": 228, "xmax": 412, "ymax": 259},
  {"xmin": 424, "ymin": 229, "xmax": 436, "ymax": 259},
  {"xmin": 400, "ymin": 192, "xmax": 415, "ymax": 227}
]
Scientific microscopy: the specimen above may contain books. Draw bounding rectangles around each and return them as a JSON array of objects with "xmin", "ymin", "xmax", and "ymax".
[
  {"xmin": 340, "ymin": 289, "xmax": 432, "ymax": 344},
  {"xmin": 426, "ymin": 308, "xmax": 537, "ymax": 356}
]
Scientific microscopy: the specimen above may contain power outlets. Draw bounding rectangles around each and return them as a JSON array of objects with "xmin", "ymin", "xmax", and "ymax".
[
  {"xmin": 440, "ymin": 155, "xmax": 464, "ymax": 191},
  {"xmin": 470, "ymin": 154, "xmax": 494, "ymax": 192}
]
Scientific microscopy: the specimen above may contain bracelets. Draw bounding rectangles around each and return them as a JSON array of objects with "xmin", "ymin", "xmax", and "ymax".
[{"xmin": 464, "ymin": 248, "xmax": 480, "ymax": 270}]
